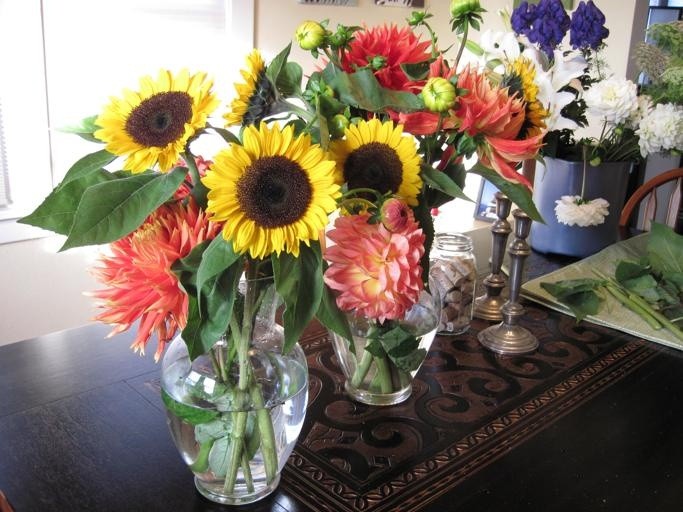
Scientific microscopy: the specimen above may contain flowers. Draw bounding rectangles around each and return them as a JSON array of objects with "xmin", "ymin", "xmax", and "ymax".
[{"xmin": 17, "ymin": 0, "xmax": 682, "ymax": 498}]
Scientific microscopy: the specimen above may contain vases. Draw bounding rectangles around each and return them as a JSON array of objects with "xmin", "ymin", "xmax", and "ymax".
[{"xmin": 155, "ymin": 273, "xmax": 310, "ymax": 505}]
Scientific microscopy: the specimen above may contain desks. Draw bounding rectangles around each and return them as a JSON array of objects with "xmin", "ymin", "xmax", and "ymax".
[{"xmin": 1, "ymin": 210, "xmax": 682, "ymax": 510}]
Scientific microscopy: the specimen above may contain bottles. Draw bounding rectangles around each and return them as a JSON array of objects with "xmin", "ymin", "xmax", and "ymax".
[{"xmin": 428, "ymin": 232, "xmax": 476, "ymax": 335}]
